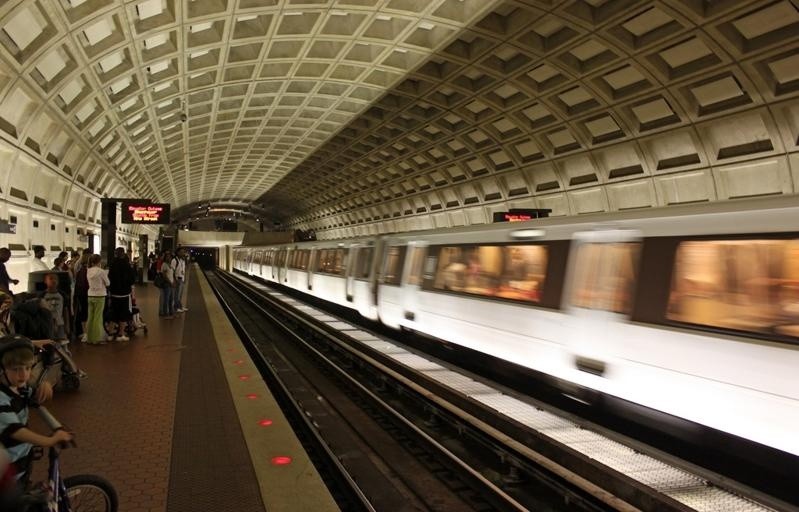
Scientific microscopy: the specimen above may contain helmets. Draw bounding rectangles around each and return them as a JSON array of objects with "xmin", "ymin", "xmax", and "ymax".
[{"xmin": 0, "ymin": 334, "xmax": 34, "ymax": 359}]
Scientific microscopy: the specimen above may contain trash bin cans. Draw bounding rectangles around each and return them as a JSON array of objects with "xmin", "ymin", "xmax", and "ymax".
[{"xmin": 27, "ymin": 270, "xmax": 73, "ymax": 339}]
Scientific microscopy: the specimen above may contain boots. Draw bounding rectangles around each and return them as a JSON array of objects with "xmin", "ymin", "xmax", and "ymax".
[{"xmin": 133, "ymin": 313, "xmax": 146, "ymax": 328}]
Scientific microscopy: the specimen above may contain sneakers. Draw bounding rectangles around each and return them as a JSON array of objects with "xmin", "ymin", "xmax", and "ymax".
[
  {"xmin": 79, "ymin": 333, "xmax": 130, "ymax": 342},
  {"xmin": 176, "ymin": 308, "xmax": 189, "ymax": 313}
]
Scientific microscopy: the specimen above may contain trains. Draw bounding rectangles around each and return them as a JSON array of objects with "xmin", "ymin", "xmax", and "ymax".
[{"xmin": 230, "ymin": 192, "xmax": 799, "ymax": 512}]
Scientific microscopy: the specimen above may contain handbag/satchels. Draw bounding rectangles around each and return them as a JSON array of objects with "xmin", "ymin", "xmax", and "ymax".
[{"xmin": 154, "ymin": 274, "xmax": 171, "ymax": 289}]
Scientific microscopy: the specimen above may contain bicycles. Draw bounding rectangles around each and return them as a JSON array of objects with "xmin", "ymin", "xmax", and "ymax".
[{"xmin": 23, "ymin": 396, "xmax": 118, "ymax": 511}]
